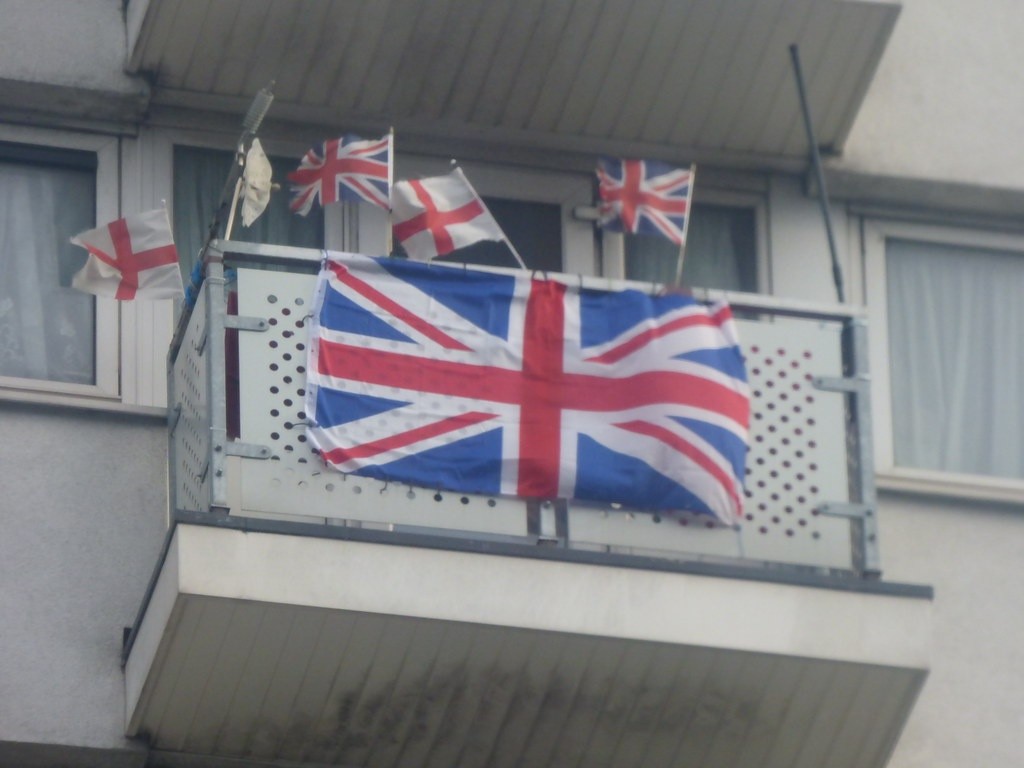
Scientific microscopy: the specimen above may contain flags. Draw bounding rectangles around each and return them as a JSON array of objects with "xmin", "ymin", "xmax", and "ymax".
[
  {"xmin": 289, "ymin": 135, "xmax": 390, "ymax": 218},
  {"xmin": 594, "ymin": 156, "xmax": 692, "ymax": 244},
  {"xmin": 393, "ymin": 168, "xmax": 504, "ymax": 261},
  {"xmin": 304, "ymin": 252, "xmax": 751, "ymax": 523},
  {"xmin": 70, "ymin": 209, "xmax": 182, "ymax": 301}
]
